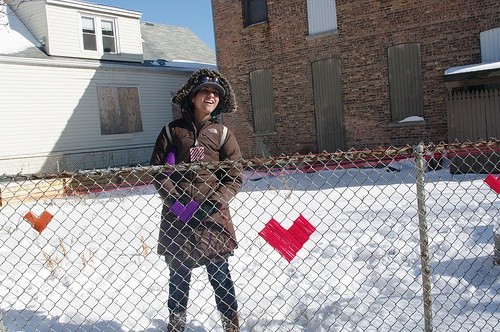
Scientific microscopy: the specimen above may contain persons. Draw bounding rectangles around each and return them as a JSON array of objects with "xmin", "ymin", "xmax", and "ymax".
[{"xmin": 150, "ymin": 71, "xmax": 243, "ymax": 332}]
